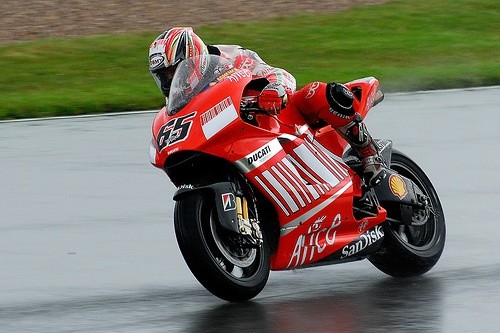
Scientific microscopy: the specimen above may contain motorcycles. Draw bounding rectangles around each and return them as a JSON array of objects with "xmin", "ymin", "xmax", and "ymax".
[{"xmin": 146, "ymin": 54, "xmax": 447, "ymax": 303}]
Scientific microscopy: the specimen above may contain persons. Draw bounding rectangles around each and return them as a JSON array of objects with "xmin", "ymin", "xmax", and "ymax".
[{"xmin": 147, "ymin": 26, "xmax": 385, "ymax": 185}]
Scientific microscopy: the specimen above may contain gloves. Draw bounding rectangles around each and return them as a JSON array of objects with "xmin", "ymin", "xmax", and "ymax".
[{"xmin": 258, "ymin": 82, "xmax": 288, "ymax": 115}]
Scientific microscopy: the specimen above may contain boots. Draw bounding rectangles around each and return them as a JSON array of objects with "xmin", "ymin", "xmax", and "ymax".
[{"xmin": 337, "ymin": 116, "xmax": 387, "ymax": 189}]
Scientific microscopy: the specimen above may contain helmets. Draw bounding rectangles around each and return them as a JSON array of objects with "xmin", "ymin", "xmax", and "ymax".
[{"xmin": 148, "ymin": 27, "xmax": 210, "ymax": 100}]
